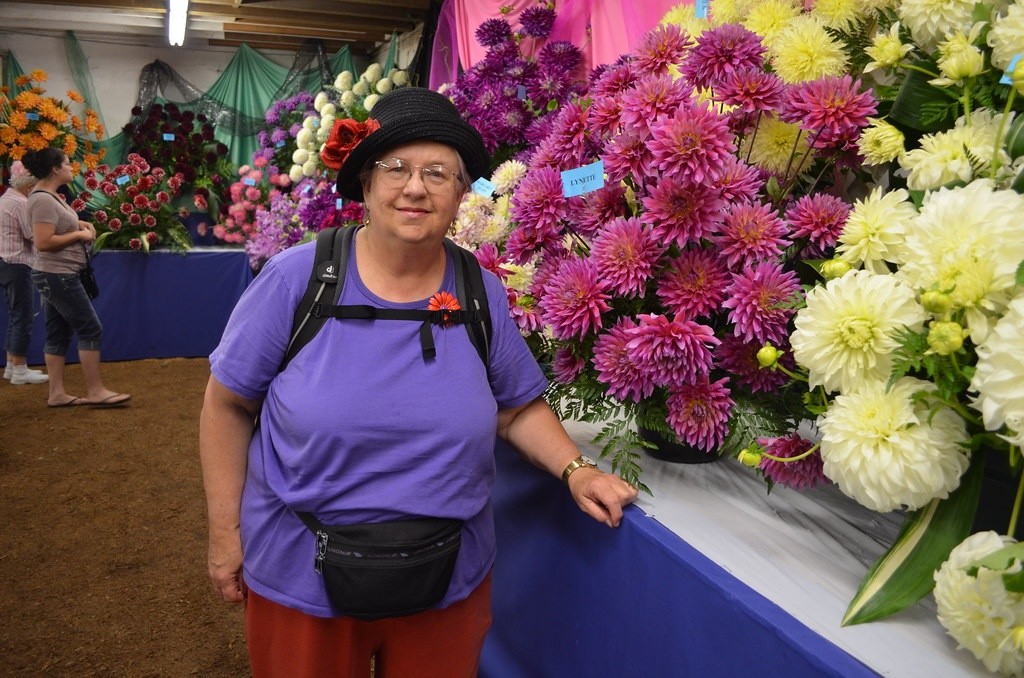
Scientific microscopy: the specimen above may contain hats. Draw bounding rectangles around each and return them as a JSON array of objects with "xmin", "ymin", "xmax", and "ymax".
[{"xmin": 321, "ymin": 86, "xmax": 491, "ymax": 203}]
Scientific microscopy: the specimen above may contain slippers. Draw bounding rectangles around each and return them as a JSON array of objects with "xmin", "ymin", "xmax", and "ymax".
[
  {"xmin": 47, "ymin": 395, "xmax": 88, "ymax": 407},
  {"xmin": 84, "ymin": 391, "xmax": 130, "ymax": 407}
]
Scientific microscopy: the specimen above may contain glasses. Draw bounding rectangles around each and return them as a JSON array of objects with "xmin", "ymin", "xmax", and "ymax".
[{"xmin": 372, "ymin": 157, "xmax": 468, "ymax": 195}]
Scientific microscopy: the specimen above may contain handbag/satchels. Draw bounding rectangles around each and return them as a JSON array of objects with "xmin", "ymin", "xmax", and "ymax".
[
  {"xmin": 79, "ymin": 265, "xmax": 99, "ymax": 301},
  {"xmin": 293, "ymin": 511, "xmax": 464, "ymax": 620}
]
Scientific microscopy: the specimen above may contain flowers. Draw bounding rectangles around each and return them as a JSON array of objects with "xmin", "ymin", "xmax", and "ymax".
[{"xmin": 0, "ymin": 1, "xmax": 1024, "ymax": 678}]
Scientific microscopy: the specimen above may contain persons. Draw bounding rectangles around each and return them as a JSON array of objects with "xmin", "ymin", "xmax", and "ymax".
[
  {"xmin": 200, "ymin": 89, "xmax": 640, "ymax": 678},
  {"xmin": 20, "ymin": 146, "xmax": 132, "ymax": 407},
  {"xmin": 0, "ymin": 160, "xmax": 49, "ymax": 385}
]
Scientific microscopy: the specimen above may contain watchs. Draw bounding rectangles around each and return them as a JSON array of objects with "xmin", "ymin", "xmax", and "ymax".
[{"xmin": 561, "ymin": 455, "xmax": 598, "ymax": 486}]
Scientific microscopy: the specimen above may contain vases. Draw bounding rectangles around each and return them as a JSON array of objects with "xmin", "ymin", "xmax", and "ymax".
[{"xmin": 638, "ymin": 390, "xmax": 728, "ymax": 464}]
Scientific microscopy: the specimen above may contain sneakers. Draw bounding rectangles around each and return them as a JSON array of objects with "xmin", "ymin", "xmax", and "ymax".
[
  {"xmin": 3, "ymin": 368, "xmax": 43, "ymax": 379},
  {"xmin": 10, "ymin": 368, "xmax": 49, "ymax": 385}
]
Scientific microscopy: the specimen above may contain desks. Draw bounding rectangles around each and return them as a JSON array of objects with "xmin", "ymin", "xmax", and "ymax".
[
  {"xmin": 476, "ymin": 393, "xmax": 1024, "ymax": 678},
  {"xmin": 0, "ymin": 245, "xmax": 253, "ymax": 367}
]
